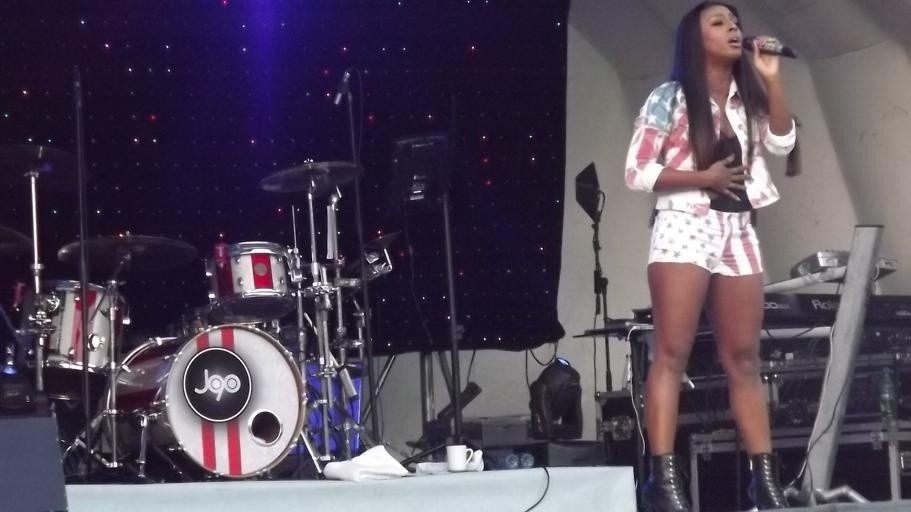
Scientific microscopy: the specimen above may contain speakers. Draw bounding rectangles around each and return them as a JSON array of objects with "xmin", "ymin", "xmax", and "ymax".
[
  {"xmin": 466, "ymin": 426, "xmax": 550, "ymax": 470},
  {"xmin": 599, "ymin": 392, "xmax": 638, "ymax": 466}
]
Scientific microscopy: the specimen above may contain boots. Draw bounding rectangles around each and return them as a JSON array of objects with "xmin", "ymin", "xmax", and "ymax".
[
  {"xmin": 744, "ymin": 451, "xmax": 790, "ymax": 510},
  {"xmin": 641, "ymin": 452, "xmax": 693, "ymax": 511}
]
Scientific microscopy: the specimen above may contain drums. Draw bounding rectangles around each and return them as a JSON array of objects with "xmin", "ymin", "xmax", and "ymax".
[
  {"xmin": 17, "ymin": 278, "xmax": 130, "ymax": 398},
  {"xmin": 101, "ymin": 325, "xmax": 307, "ymax": 478},
  {"xmin": 0, "ymin": 227, "xmax": 34, "ymax": 312},
  {"xmin": 205, "ymin": 242, "xmax": 298, "ymax": 324}
]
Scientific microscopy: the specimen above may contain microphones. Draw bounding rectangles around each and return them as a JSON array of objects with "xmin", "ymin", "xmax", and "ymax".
[
  {"xmin": 332, "ymin": 61, "xmax": 357, "ymax": 107},
  {"xmin": 742, "ymin": 37, "xmax": 797, "ymax": 59}
]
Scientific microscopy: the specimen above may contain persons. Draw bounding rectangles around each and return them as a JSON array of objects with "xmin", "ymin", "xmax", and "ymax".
[{"xmin": 623, "ymin": 0, "xmax": 798, "ymax": 512}]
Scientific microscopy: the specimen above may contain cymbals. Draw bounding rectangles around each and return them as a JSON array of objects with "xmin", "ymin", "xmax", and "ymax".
[
  {"xmin": 258, "ymin": 161, "xmax": 363, "ymax": 194},
  {"xmin": 56, "ymin": 235, "xmax": 198, "ymax": 277},
  {"xmin": 2, "ymin": 143, "xmax": 78, "ymax": 182}
]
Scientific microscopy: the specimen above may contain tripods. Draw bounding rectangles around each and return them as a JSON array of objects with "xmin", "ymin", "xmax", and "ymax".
[
  {"xmin": 404, "ymin": 190, "xmax": 503, "ymax": 470},
  {"xmin": 361, "ymin": 352, "xmax": 463, "ymax": 438},
  {"xmin": 63, "ymin": 279, "xmax": 190, "ymax": 481}
]
